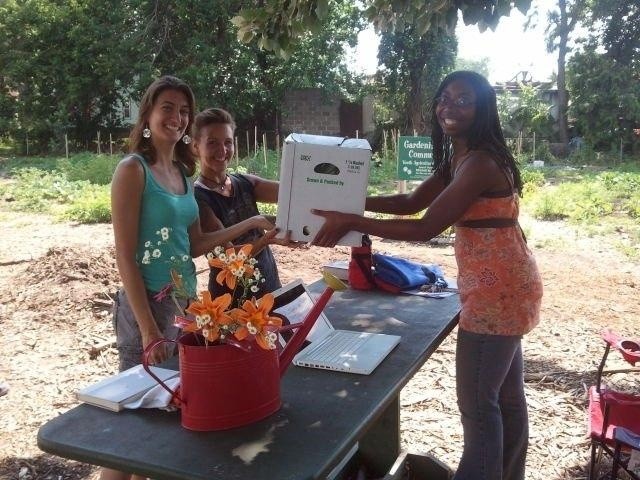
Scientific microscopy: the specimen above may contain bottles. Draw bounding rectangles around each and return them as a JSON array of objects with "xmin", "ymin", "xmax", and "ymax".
[{"xmin": 348, "ymin": 235, "xmax": 375, "ymax": 291}]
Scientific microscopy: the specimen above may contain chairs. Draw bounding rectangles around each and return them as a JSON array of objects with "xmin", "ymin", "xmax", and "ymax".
[{"xmin": 588, "ymin": 331, "xmax": 640, "ymax": 480}]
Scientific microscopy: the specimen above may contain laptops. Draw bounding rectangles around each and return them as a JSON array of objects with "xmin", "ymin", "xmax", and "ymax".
[{"xmin": 255, "ymin": 278, "xmax": 402, "ymax": 375}]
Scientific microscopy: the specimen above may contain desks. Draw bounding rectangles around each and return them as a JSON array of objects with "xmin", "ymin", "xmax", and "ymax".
[{"xmin": 36, "ymin": 278, "xmax": 462, "ymax": 480}]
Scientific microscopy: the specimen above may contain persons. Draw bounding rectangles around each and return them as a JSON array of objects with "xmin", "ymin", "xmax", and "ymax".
[
  {"xmin": 311, "ymin": 68, "xmax": 545, "ymax": 478},
  {"xmin": 109, "ymin": 74, "xmax": 276, "ymax": 373},
  {"xmin": 192, "ymin": 106, "xmax": 311, "ymax": 311}
]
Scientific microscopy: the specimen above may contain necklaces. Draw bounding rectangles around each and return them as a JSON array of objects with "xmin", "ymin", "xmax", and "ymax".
[{"xmin": 199, "ymin": 172, "xmax": 229, "ymax": 190}]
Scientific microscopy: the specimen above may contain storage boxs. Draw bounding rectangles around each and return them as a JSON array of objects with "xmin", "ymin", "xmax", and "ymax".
[{"xmin": 275, "ymin": 131, "xmax": 373, "ymax": 247}]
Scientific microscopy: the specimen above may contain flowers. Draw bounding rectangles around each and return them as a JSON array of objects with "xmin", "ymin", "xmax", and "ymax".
[{"xmin": 142, "ymin": 227, "xmax": 282, "ymax": 350}]
